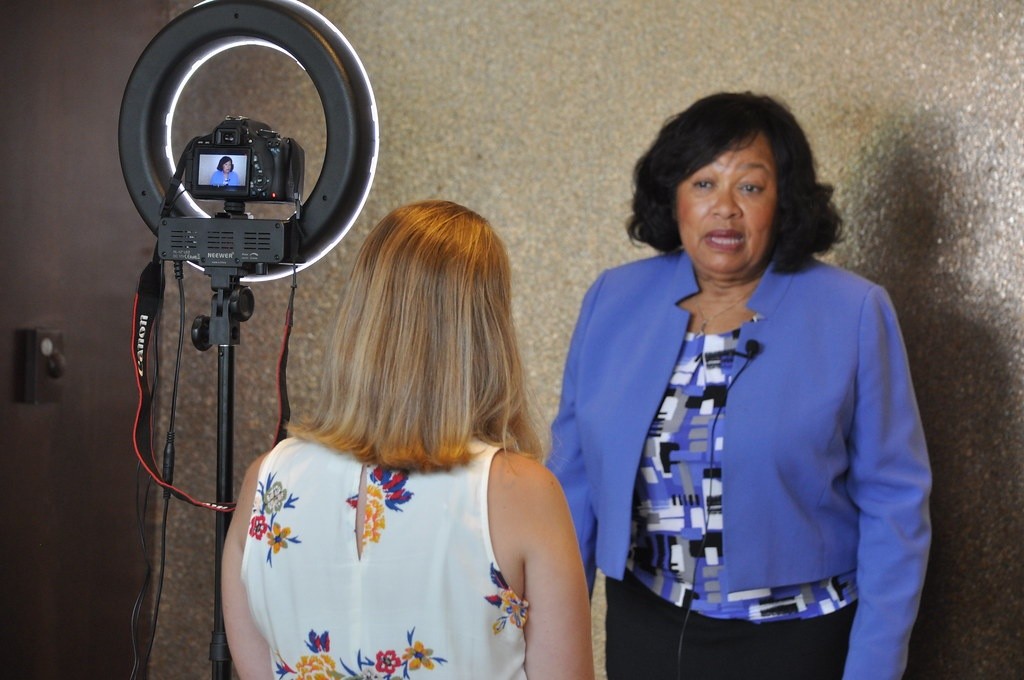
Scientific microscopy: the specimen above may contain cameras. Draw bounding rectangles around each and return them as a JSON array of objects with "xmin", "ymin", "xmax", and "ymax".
[{"xmin": 184, "ymin": 115, "xmax": 305, "ymax": 204}]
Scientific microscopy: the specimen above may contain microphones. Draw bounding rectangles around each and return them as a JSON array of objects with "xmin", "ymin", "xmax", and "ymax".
[{"xmin": 744, "ymin": 339, "xmax": 759, "ymax": 361}]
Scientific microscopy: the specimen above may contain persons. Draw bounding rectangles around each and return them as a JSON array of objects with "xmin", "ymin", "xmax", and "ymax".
[
  {"xmin": 210, "ymin": 157, "xmax": 238, "ymax": 186},
  {"xmin": 221, "ymin": 201, "xmax": 595, "ymax": 680},
  {"xmin": 546, "ymin": 91, "xmax": 934, "ymax": 680}
]
